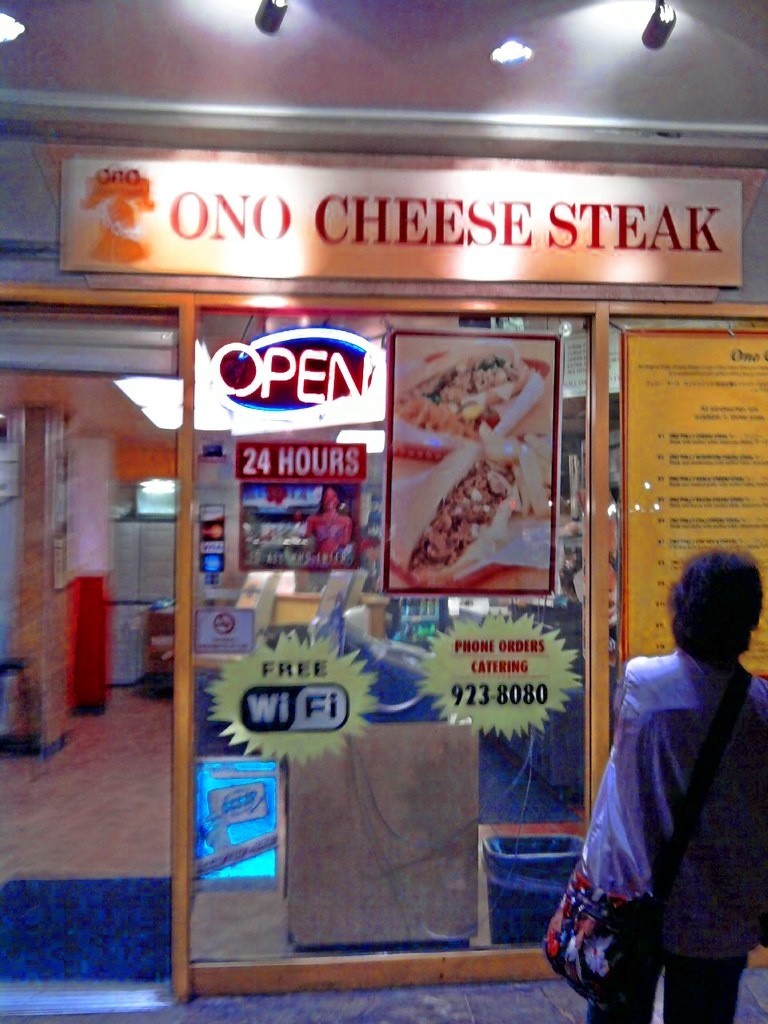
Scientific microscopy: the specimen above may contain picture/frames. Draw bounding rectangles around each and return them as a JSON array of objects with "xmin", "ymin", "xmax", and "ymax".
[{"xmin": 239, "ymin": 482, "xmax": 360, "ymax": 572}]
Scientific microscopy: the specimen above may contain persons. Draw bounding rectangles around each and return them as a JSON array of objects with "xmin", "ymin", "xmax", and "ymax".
[
  {"xmin": 357, "ymin": 537, "xmax": 400, "ymax": 635},
  {"xmin": 582, "ymin": 549, "xmax": 768, "ymax": 1024},
  {"xmin": 304, "ymin": 486, "xmax": 352, "ymax": 569}
]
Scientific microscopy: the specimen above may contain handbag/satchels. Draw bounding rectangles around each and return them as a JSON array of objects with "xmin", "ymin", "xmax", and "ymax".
[{"xmin": 543, "ymin": 860, "xmax": 652, "ymax": 1002}]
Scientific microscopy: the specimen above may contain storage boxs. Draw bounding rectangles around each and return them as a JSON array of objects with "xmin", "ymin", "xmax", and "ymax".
[{"xmin": 275, "ymin": 715, "xmax": 480, "ymax": 947}]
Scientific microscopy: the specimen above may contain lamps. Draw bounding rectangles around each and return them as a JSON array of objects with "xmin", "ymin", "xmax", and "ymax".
[
  {"xmin": 640, "ymin": 0, "xmax": 677, "ymax": 50},
  {"xmin": 254, "ymin": 0, "xmax": 288, "ymax": 35}
]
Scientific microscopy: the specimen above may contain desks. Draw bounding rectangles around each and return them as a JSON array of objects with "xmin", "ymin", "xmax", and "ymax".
[{"xmin": 269, "ymin": 593, "xmax": 391, "ymax": 640}]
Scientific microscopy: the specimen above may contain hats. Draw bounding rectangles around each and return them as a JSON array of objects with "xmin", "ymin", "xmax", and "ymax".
[{"xmin": 671, "ymin": 552, "xmax": 762, "ymax": 638}]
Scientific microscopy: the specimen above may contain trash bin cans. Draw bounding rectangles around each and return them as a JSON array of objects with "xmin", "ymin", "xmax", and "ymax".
[
  {"xmin": 1, "ymin": 658, "xmax": 47, "ymax": 756},
  {"xmin": 481, "ymin": 834, "xmax": 585, "ymax": 944}
]
